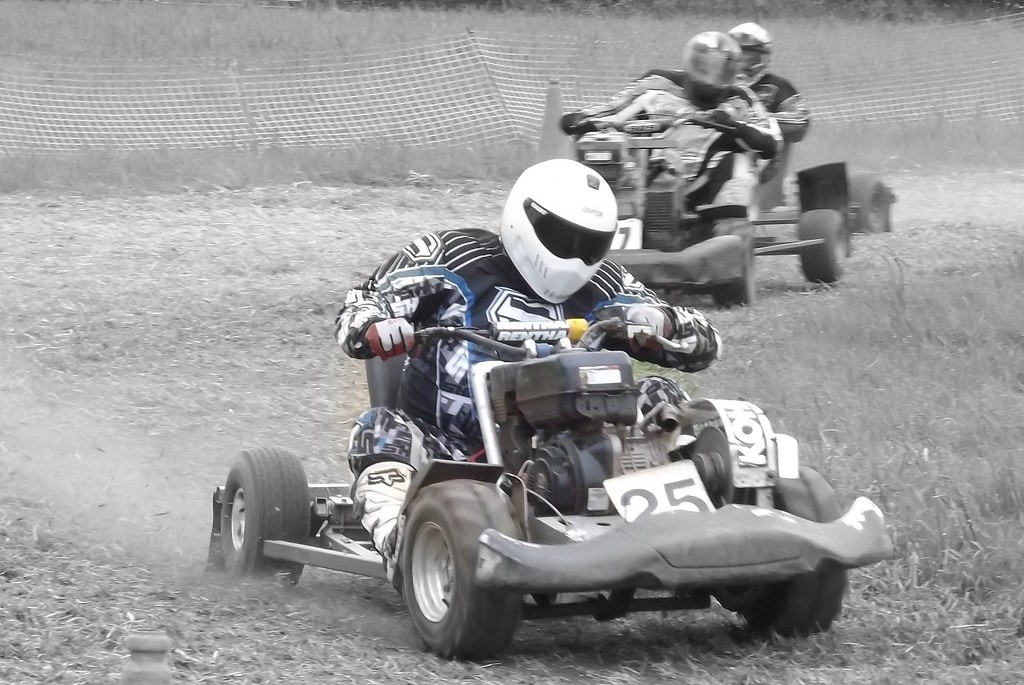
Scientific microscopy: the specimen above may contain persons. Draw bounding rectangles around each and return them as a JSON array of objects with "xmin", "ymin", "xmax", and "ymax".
[
  {"xmin": 641, "ymin": 21, "xmax": 809, "ymax": 188},
  {"xmin": 335, "ymin": 157, "xmax": 721, "ymax": 593},
  {"xmin": 559, "ymin": 31, "xmax": 784, "ymax": 219}
]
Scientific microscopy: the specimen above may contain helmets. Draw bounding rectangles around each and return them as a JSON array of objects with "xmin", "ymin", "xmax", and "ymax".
[
  {"xmin": 685, "ymin": 33, "xmax": 742, "ymax": 90},
  {"xmin": 499, "ymin": 160, "xmax": 617, "ymax": 302},
  {"xmin": 728, "ymin": 24, "xmax": 774, "ymax": 88}
]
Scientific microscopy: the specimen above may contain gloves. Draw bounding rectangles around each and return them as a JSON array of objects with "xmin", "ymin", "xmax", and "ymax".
[
  {"xmin": 627, "ymin": 306, "xmax": 666, "ymax": 350},
  {"xmin": 365, "ymin": 317, "xmax": 422, "ymax": 363},
  {"xmin": 560, "ymin": 111, "xmax": 590, "ymax": 135},
  {"xmin": 705, "ymin": 112, "xmax": 732, "ymax": 135}
]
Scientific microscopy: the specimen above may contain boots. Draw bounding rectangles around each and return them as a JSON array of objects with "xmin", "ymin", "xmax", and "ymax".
[{"xmin": 348, "ymin": 458, "xmax": 407, "ymax": 594}]
